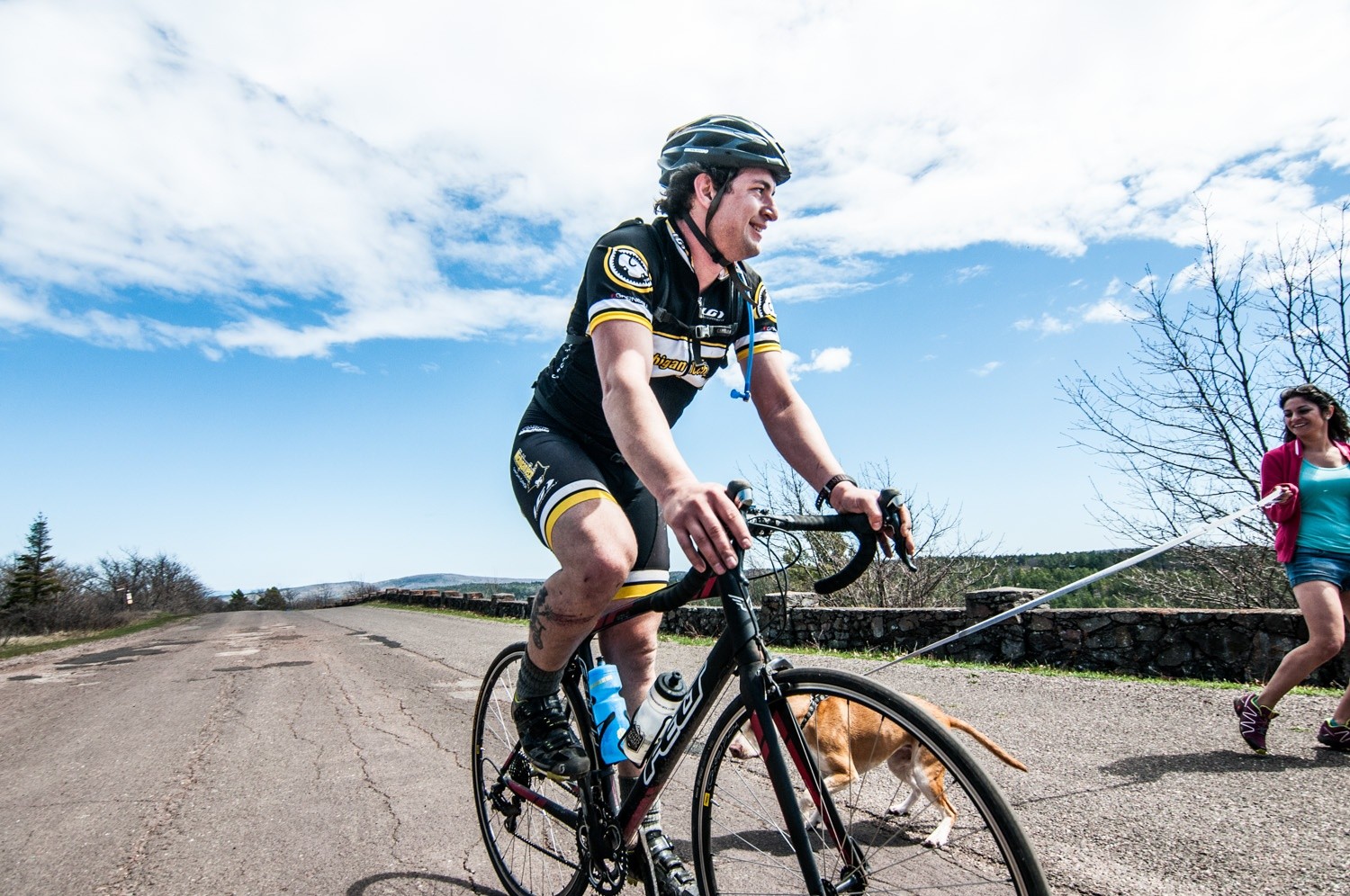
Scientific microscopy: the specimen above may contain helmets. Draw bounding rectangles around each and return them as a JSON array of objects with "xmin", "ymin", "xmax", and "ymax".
[{"xmin": 657, "ymin": 113, "xmax": 792, "ymax": 192}]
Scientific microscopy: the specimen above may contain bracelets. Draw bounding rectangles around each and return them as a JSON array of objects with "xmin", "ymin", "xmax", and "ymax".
[{"xmin": 816, "ymin": 475, "xmax": 859, "ymax": 513}]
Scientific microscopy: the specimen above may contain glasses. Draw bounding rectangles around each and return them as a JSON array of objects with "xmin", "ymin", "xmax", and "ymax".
[{"xmin": 1279, "ymin": 384, "xmax": 1325, "ymax": 400}]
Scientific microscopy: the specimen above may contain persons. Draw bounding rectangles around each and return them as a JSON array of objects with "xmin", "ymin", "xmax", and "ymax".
[
  {"xmin": 509, "ymin": 115, "xmax": 916, "ymax": 896},
  {"xmin": 1232, "ymin": 386, "xmax": 1350, "ymax": 754}
]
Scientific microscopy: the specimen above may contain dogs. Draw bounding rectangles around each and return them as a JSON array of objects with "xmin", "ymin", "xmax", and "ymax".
[{"xmin": 728, "ymin": 689, "xmax": 1030, "ymax": 848}]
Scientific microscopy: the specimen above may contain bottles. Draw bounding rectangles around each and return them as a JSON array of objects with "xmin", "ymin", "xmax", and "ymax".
[
  {"xmin": 623, "ymin": 671, "xmax": 688, "ymax": 763},
  {"xmin": 586, "ymin": 654, "xmax": 635, "ymax": 764}
]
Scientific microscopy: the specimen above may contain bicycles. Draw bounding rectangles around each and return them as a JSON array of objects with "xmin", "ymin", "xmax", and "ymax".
[{"xmin": 470, "ymin": 479, "xmax": 1052, "ymax": 896}]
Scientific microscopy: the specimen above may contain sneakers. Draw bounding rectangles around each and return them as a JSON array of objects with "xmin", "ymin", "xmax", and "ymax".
[
  {"xmin": 510, "ymin": 688, "xmax": 591, "ymax": 781},
  {"xmin": 1317, "ymin": 719, "xmax": 1350, "ymax": 750},
  {"xmin": 625, "ymin": 829, "xmax": 699, "ymax": 896},
  {"xmin": 1234, "ymin": 693, "xmax": 1280, "ymax": 755}
]
